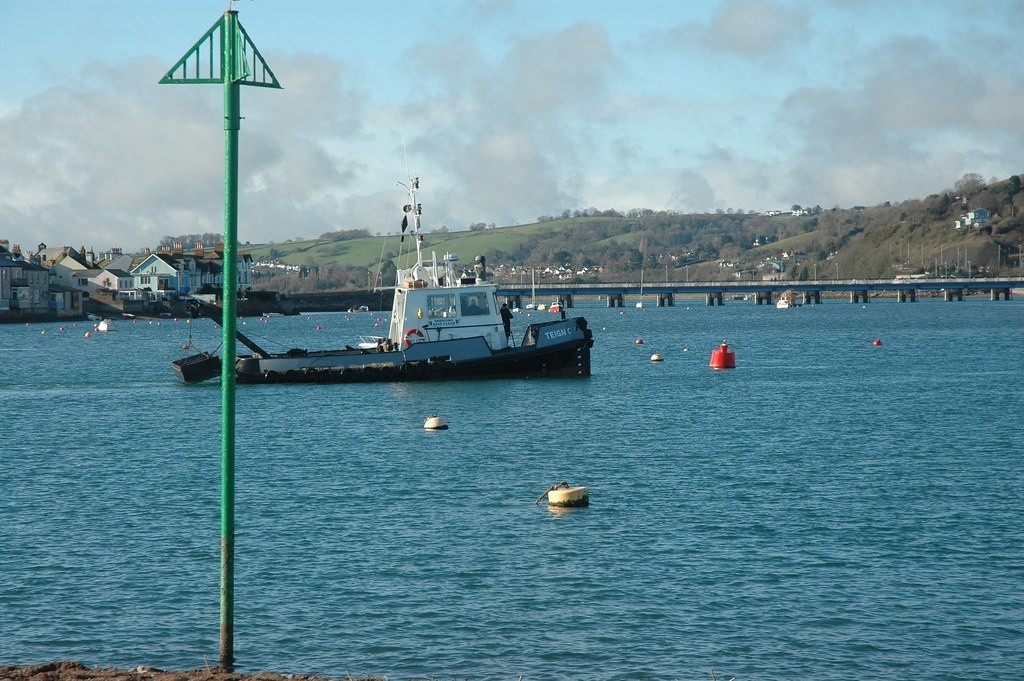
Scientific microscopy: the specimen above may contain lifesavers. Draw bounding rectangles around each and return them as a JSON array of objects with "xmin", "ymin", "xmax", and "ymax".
[{"xmin": 402, "ymin": 328, "xmax": 425, "ymax": 348}]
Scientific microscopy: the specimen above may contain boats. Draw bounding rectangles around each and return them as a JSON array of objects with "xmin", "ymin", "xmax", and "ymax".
[{"xmin": 169, "ymin": 174, "xmax": 596, "ymax": 385}]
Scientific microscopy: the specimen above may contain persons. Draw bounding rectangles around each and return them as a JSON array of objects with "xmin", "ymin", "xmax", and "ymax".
[
  {"xmin": 468, "ymin": 299, "xmax": 482, "ymax": 315},
  {"xmin": 500, "ymin": 303, "xmax": 514, "ymax": 346}
]
